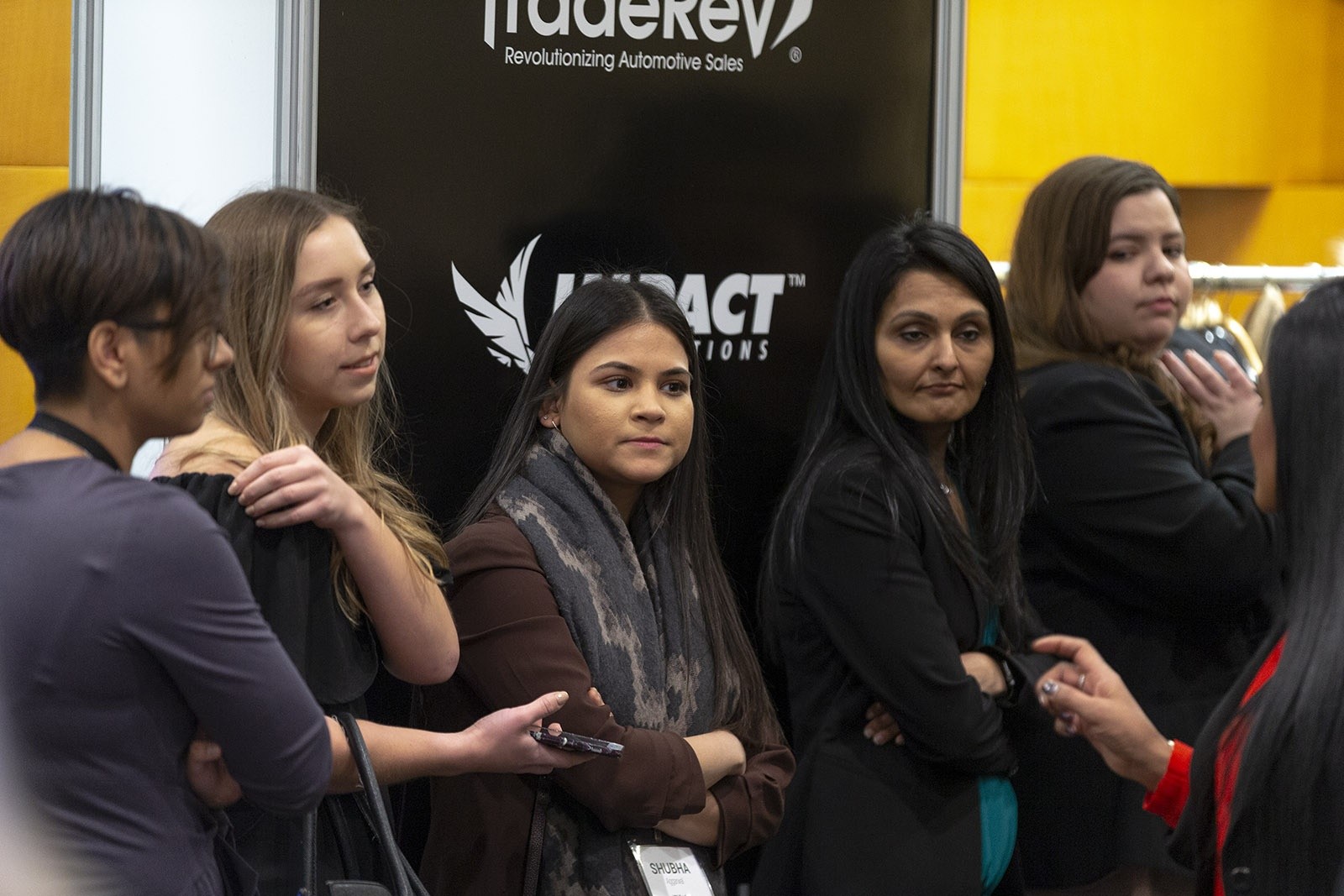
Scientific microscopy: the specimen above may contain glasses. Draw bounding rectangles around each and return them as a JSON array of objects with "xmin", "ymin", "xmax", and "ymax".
[{"xmin": 112, "ymin": 305, "xmax": 225, "ymax": 342}]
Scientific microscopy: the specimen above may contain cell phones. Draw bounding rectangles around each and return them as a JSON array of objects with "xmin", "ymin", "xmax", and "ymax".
[{"xmin": 528, "ymin": 725, "xmax": 627, "ymax": 760}]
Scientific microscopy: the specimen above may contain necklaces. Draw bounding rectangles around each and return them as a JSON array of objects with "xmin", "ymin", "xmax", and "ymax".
[{"xmin": 941, "ymin": 484, "xmax": 956, "ymax": 494}]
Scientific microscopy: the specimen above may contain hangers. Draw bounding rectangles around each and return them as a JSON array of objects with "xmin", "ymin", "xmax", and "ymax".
[{"xmin": 1169, "ymin": 272, "xmax": 1289, "ymax": 375}]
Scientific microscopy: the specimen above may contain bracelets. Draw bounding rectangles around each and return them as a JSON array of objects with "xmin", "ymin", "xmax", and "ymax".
[{"xmin": 1167, "ymin": 739, "xmax": 1175, "ymax": 752}]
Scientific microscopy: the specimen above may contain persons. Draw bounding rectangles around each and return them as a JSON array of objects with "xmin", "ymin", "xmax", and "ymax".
[
  {"xmin": 753, "ymin": 216, "xmax": 1071, "ymax": 896},
  {"xmin": 1031, "ymin": 274, "xmax": 1344, "ymax": 896},
  {"xmin": 413, "ymin": 279, "xmax": 797, "ymax": 896},
  {"xmin": 142, "ymin": 188, "xmax": 603, "ymax": 896},
  {"xmin": 1006, "ymin": 155, "xmax": 1265, "ymax": 896},
  {"xmin": 0, "ymin": 189, "xmax": 334, "ymax": 896}
]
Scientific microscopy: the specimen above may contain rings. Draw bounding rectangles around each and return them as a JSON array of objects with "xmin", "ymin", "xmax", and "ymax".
[{"xmin": 1077, "ymin": 674, "xmax": 1085, "ymax": 690}]
